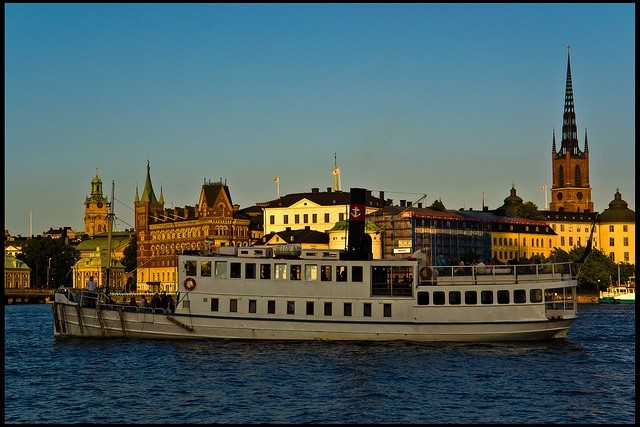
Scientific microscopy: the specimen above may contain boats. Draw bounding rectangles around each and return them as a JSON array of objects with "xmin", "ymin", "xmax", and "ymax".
[{"xmin": 599, "ymin": 271, "xmax": 636, "ymax": 303}]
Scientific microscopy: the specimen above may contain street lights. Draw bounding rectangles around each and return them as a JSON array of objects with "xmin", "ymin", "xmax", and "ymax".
[{"xmin": 46, "ymin": 257, "xmax": 52, "ymax": 289}]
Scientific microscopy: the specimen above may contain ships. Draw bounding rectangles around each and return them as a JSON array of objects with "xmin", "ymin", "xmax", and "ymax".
[{"xmin": 50, "ymin": 187, "xmax": 601, "ymax": 343}]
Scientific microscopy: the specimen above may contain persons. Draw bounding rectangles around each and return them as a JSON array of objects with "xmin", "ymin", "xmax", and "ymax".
[
  {"xmin": 139, "ymin": 296, "xmax": 148, "ymax": 312},
  {"xmin": 394, "ymin": 275, "xmax": 413, "ymax": 294},
  {"xmin": 129, "ymin": 297, "xmax": 138, "ymax": 308},
  {"xmin": 511, "ymin": 257, "xmax": 531, "ymax": 274},
  {"xmin": 436, "ymin": 255, "xmax": 452, "ymax": 276},
  {"xmin": 455, "ymin": 256, "xmax": 465, "ymax": 266},
  {"xmin": 455, "ymin": 261, "xmax": 471, "ymax": 275},
  {"xmin": 189, "ymin": 262, "xmax": 194, "ymax": 275},
  {"xmin": 337, "ymin": 270, "xmax": 346, "ymax": 280},
  {"xmin": 85, "ymin": 276, "xmax": 96, "ymax": 307},
  {"xmin": 151, "ymin": 291, "xmax": 174, "ymax": 314},
  {"xmin": 121, "ymin": 297, "xmax": 126, "ymax": 310},
  {"xmin": 471, "ymin": 260, "xmax": 477, "ymax": 266}
]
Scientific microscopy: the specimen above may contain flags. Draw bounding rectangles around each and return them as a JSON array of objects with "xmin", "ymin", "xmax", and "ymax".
[
  {"xmin": 332, "ymin": 167, "xmax": 340, "ymax": 176},
  {"xmin": 540, "ymin": 186, "xmax": 545, "ymax": 193}
]
